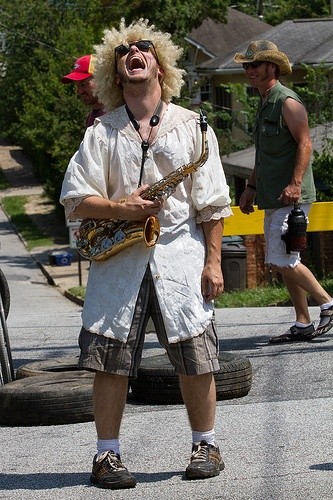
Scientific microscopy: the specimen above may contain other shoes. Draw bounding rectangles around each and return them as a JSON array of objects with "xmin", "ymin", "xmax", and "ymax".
[
  {"xmin": 269, "ymin": 323, "xmax": 315, "ymax": 343},
  {"xmin": 311, "ymin": 304, "xmax": 333, "ymax": 337}
]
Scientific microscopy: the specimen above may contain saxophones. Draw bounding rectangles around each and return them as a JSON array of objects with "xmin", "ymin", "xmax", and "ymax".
[{"xmin": 75, "ymin": 106, "xmax": 209, "ymax": 262}]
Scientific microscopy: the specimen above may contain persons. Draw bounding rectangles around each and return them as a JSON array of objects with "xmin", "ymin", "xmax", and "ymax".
[
  {"xmin": 233, "ymin": 38, "xmax": 333, "ymax": 342},
  {"xmin": 63, "ymin": 55, "xmax": 107, "ymax": 128},
  {"xmin": 59, "ymin": 18, "xmax": 233, "ymax": 487}
]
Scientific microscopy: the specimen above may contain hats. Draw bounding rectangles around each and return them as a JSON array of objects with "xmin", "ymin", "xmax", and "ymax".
[
  {"xmin": 62, "ymin": 54, "xmax": 100, "ymax": 83},
  {"xmin": 233, "ymin": 40, "xmax": 294, "ymax": 75}
]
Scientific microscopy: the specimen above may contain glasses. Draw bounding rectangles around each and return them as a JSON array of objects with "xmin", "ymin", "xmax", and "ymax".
[
  {"xmin": 243, "ymin": 61, "xmax": 267, "ymax": 69},
  {"xmin": 113, "ymin": 39, "xmax": 160, "ymax": 73}
]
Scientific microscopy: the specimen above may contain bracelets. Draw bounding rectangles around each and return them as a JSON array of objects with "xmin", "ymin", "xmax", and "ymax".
[{"xmin": 246, "ymin": 184, "xmax": 256, "ymax": 190}]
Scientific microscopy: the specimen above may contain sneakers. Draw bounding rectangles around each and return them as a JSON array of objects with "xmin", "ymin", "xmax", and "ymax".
[
  {"xmin": 89, "ymin": 450, "xmax": 136, "ymax": 490},
  {"xmin": 185, "ymin": 440, "xmax": 226, "ymax": 478}
]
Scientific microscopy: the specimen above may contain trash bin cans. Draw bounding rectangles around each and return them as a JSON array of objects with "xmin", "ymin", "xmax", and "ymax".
[
  {"xmin": 221, "ymin": 243, "xmax": 248, "ymax": 289},
  {"xmin": 222, "ymin": 235, "xmax": 243, "ymax": 245},
  {"xmin": 67, "ymin": 218, "xmax": 83, "ymax": 249}
]
